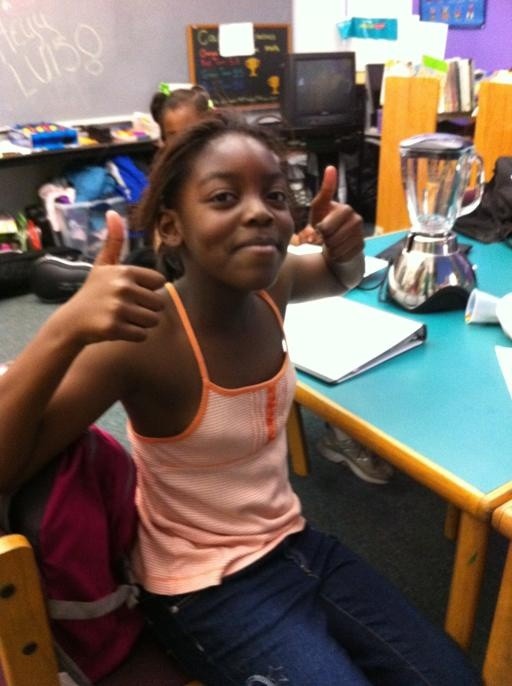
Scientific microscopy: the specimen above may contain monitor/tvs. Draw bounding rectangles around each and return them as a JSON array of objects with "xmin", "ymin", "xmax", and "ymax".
[{"xmin": 280, "ymin": 52, "xmax": 356, "ymax": 128}]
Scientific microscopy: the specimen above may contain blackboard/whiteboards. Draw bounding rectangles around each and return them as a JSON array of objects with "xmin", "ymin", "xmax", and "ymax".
[{"xmin": 188, "ymin": 23, "xmax": 293, "ymax": 112}]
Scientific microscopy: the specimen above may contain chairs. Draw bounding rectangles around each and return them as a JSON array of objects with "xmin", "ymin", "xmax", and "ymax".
[{"xmin": 0, "ymin": 521, "xmax": 204, "ymax": 686}]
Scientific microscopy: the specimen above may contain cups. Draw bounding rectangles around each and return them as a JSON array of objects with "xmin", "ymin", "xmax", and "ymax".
[{"xmin": 464, "ymin": 288, "xmax": 502, "ymax": 326}]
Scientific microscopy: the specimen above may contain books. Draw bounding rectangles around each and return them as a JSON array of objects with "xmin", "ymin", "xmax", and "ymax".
[
  {"xmin": 279, "ymin": 241, "xmax": 390, "ymax": 285},
  {"xmin": 380, "ymin": 54, "xmax": 475, "ymax": 116}
]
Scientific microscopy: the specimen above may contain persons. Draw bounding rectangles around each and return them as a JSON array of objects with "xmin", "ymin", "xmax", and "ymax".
[
  {"xmin": 141, "ymin": 84, "xmax": 215, "ymax": 277},
  {"xmin": 0, "ymin": 109, "xmax": 488, "ymax": 686}
]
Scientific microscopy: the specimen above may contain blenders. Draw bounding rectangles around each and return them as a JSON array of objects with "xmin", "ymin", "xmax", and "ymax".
[{"xmin": 384, "ymin": 132, "xmax": 485, "ymax": 315}]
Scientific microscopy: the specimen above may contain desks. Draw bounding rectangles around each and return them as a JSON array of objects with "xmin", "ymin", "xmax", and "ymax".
[{"xmin": 286, "ymin": 227, "xmax": 512, "ymax": 686}]
